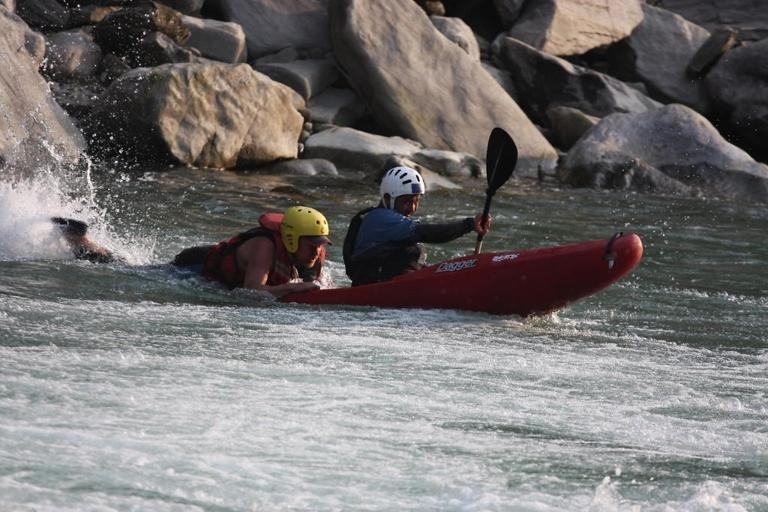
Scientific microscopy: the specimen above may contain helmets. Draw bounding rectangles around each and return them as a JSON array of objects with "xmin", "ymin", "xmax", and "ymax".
[
  {"xmin": 279, "ymin": 205, "xmax": 330, "ymax": 254},
  {"xmin": 380, "ymin": 166, "xmax": 425, "ymax": 199}
]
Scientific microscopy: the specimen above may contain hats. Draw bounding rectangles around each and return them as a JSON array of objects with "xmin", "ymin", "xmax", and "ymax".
[{"xmin": 302, "ymin": 236, "xmax": 334, "ymax": 247}]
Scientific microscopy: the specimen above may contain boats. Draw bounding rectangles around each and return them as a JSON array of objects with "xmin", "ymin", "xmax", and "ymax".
[{"xmin": 277, "ymin": 232, "xmax": 643, "ymax": 318}]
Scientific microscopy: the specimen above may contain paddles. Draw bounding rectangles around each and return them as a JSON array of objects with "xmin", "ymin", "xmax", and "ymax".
[{"xmin": 474, "ymin": 127, "xmax": 518, "ymax": 255}]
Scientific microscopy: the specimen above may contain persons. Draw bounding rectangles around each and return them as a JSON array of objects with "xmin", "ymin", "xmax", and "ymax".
[
  {"xmin": 343, "ymin": 166, "xmax": 492, "ymax": 287},
  {"xmin": 50, "ymin": 205, "xmax": 333, "ymax": 299}
]
